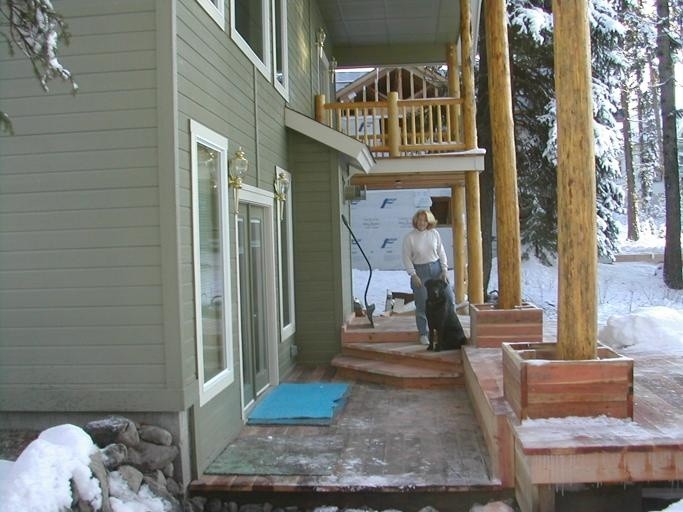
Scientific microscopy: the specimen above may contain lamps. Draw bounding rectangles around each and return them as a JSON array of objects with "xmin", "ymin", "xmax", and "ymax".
[
  {"xmin": 314, "ymin": 27, "xmax": 327, "ymax": 47},
  {"xmin": 273, "ymin": 170, "xmax": 290, "ymax": 201},
  {"xmin": 228, "ymin": 145, "xmax": 248, "ymax": 188}
]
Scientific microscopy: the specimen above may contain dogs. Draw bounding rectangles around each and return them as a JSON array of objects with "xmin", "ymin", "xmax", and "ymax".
[{"xmin": 423, "ymin": 278, "xmax": 467, "ymax": 352}]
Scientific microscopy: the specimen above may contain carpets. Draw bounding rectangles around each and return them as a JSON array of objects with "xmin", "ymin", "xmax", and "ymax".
[{"xmin": 203, "ymin": 383, "xmax": 353, "ymax": 476}]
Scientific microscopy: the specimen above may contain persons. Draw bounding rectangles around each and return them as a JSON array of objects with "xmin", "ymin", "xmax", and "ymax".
[{"xmin": 401, "ymin": 207, "xmax": 450, "ymax": 345}]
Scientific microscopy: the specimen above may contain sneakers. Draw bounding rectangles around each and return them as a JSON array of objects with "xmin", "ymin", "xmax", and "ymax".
[{"xmin": 419, "ymin": 334, "xmax": 429, "ymax": 345}]
